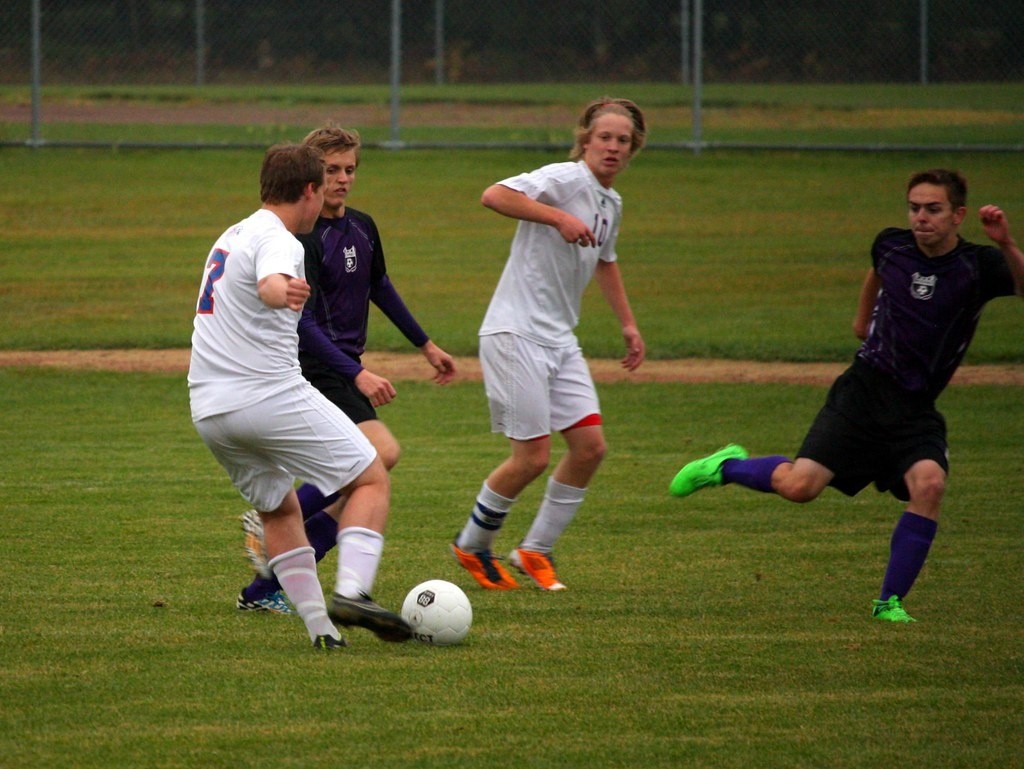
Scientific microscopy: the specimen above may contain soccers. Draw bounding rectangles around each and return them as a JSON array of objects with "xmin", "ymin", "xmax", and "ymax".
[{"xmin": 400, "ymin": 579, "xmax": 473, "ymax": 647}]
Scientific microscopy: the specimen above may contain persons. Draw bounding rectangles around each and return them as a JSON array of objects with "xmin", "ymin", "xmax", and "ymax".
[
  {"xmin": 188, "ymin": 127, "xmax": 459, "ymax": 650},
  {"xmin": 671, "ymin": 168, "xmax": 1024, "ymax": 626},
  {"xmin": 451, "ymin": 99, "xmax": 645, "ymax": 596}
]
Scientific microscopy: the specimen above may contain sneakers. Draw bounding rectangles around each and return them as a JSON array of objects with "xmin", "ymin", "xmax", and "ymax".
[
  {"xmin": 448, "ymin": 531, "xmax": 522, "ymax": 590},
  {"xmin": 236, "ymin": 585, "xmax": 298, "ymax": 615},
  {"xmin": 311, "ymin": 633, "xmax": 346, "ymax": 652},
  {"xmin": 239, "ymin": 508, "xmax": 271, "ymax": 580},
  {"xmin": 327, "ymin": 590, "xmax": 414, "ymax": 643},
  {"xmin": 669, "ymin": 441, "xmax": 751, "ymax": 499},
  {"xmin": 508, "ymin": 539, "xmax": 570, "ymax": 593},
  {"xmin": 871, "ymin": 596, "xmax": 916, "ymax": 623}
]
